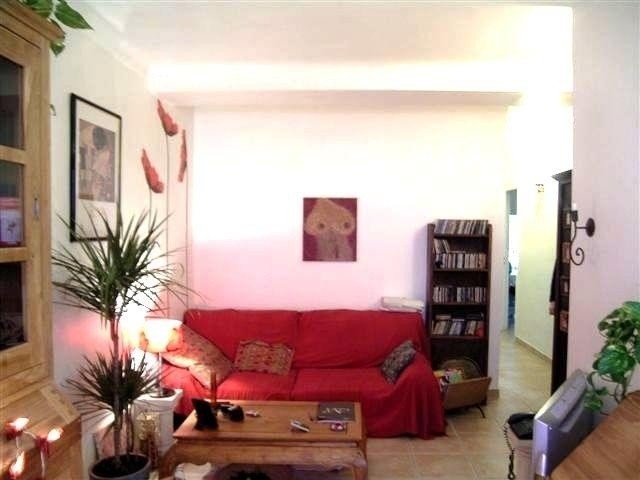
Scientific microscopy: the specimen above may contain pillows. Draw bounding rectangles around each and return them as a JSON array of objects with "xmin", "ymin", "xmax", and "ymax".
[
  {"xmin": 380, "ymin": 340, "xmax": 416, "ymax": 385},
  {"xmin": 233, "ymin": 339, "xmax": 296, "ymax": 376},
  {"xmin": 162, "ymin": 324, "xmax": 233, "ymax": 391}
]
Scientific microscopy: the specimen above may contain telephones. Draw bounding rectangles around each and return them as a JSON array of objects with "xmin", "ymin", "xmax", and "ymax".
[{"xmin": 507, "ymin": 413, "xmax": 535, "ymax": 480}]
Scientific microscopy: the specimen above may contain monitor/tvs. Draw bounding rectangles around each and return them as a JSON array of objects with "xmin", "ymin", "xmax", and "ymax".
[{"xmin": 530, "ymin": 368, "xmax": 597, "ymax": 480}]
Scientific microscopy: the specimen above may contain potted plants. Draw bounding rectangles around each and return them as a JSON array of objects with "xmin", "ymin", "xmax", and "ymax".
[{"xmin": 51, "ymin": 201, "xmax": 207, "ymax": 480}]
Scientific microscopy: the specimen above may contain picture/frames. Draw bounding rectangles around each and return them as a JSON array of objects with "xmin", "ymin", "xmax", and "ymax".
[{"xmin": 69, "ymin": 91, "xmax": 122, "ymax": 242}]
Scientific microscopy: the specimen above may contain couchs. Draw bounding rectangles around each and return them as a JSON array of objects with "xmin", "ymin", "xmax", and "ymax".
[{"xmin": 157, "ymin": 308, "xmax": 434, "ymax": 440}]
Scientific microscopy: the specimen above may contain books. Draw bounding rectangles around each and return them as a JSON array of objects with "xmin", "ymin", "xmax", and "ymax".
[
  {"xmin": 432, "ymin": 238, "xmax": 487, "ymax": 269},
  {"xmin": 431, "ymin": 313, "xmax": 487, "ymax": 337},
  {"xmin": 316, "ymin": 401, "xmax": 355, "ymax": 423},
  {"xmin": 433, "ymin": 218, "xmax": 491, "ymax": 235},
  {"xmin": 432, "ymin": 284, "xmax": 486, "ymax": 303}
]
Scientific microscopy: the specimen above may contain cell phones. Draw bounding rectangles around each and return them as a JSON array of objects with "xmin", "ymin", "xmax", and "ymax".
[{"xmin": 246, "ymin": 409, "xmax": 262, "ymax": 417}]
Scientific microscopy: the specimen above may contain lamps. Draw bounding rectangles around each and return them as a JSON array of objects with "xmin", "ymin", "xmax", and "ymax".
[{"xmin": 138, "ymin": 319, "xmax": 183, "ymax": 398}]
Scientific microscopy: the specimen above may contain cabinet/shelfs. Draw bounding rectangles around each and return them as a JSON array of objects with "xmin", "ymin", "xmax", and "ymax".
[
  {"xmin": 551, "ymin": 168, "xmax": 572, "ymax": 399},
  {"xmin": 0, "ymin": 0, "xmax": 85, "ymax": 480},
  {"xmin": 425, "ymin": 223, "xmax": 493, "ymax": 405}
]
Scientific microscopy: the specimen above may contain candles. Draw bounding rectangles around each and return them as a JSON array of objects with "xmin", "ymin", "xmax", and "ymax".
[
  {"xmin": 210, "ymin": 370, "xmax": 217, "ymax": 410},
  {"xmin": 571, "ymin": 202, "xmax": 577, "ymax": 211}
]
